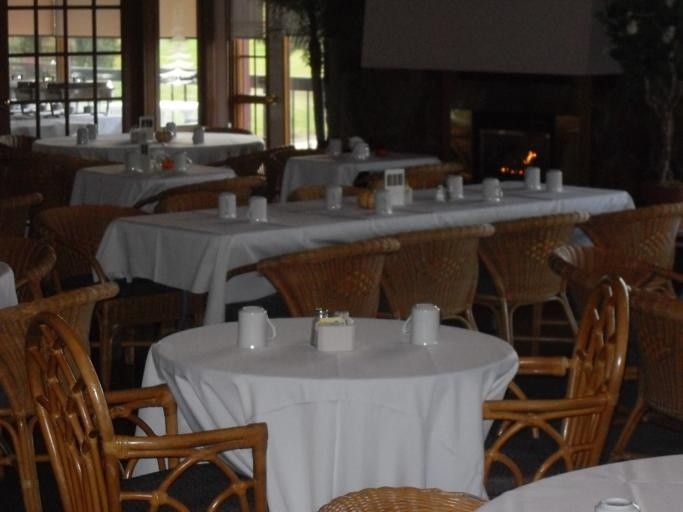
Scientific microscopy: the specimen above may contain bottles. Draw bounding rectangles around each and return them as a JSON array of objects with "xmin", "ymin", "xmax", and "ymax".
[
  {"xmin": 431, "ymin": 184, "xmax": 444, "ymax": 203},
  {"xmin": 309, "ymin": 308, "xmax": 350, "ymax": 347},
  {"xmin": 404, "ymin": 182, "xmax": 412, "ymax": 205}
]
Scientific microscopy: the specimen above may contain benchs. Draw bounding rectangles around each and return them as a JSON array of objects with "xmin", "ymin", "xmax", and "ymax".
[{"xmin": 11, "ymin": 79, "xmax": 120, "ymax": 116}]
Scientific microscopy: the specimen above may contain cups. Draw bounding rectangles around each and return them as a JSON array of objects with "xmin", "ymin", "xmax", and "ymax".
[
  {"xmin": 216, "ymin": 192, "xmax": 239, "ymax": 222},
  {"xmin": 544, "ymin": 168, "xmax": 565, "ymax": 194},
  {"xmin": 73, "ymin": 121, "xmax": 204, "ymax": 145},
  {"xmin": 400, "ymin": 298, "xmax": 440, "ymax": 349},
  {"xmin": 234, "ymin": 304, "xmax": 275, "ymax": 352},
  {"xmin": 323, "ymin": 183, "xmax": 345, "ymax": 210},
  {"xmin": 481, "ymin": 176, "xmax": 503, "ymax": 203},
  {"xmin": 244, "ymin": 194, "xmax": 270, "ymax": 224},
  {"xmin": 523, "ymin": 166, "xmax": 541, "ymax": 190},
  {"xmin": 446, "ymin": 172, "xmax": 464, "ymax": 201},
  {"xmin": 374, "ymin": 187, "xmax": 393, "ymax": 217},
  {"xmin": 116, "ymin": 146, "xmax": 194, "ymax": 177},
  {"xmin": 324, "ymin": 134, "xmax": 368, "ymax": 161},
  {"xmin": 592, "ymin": 496, "xmax": 641, "ymax": 512}
]
